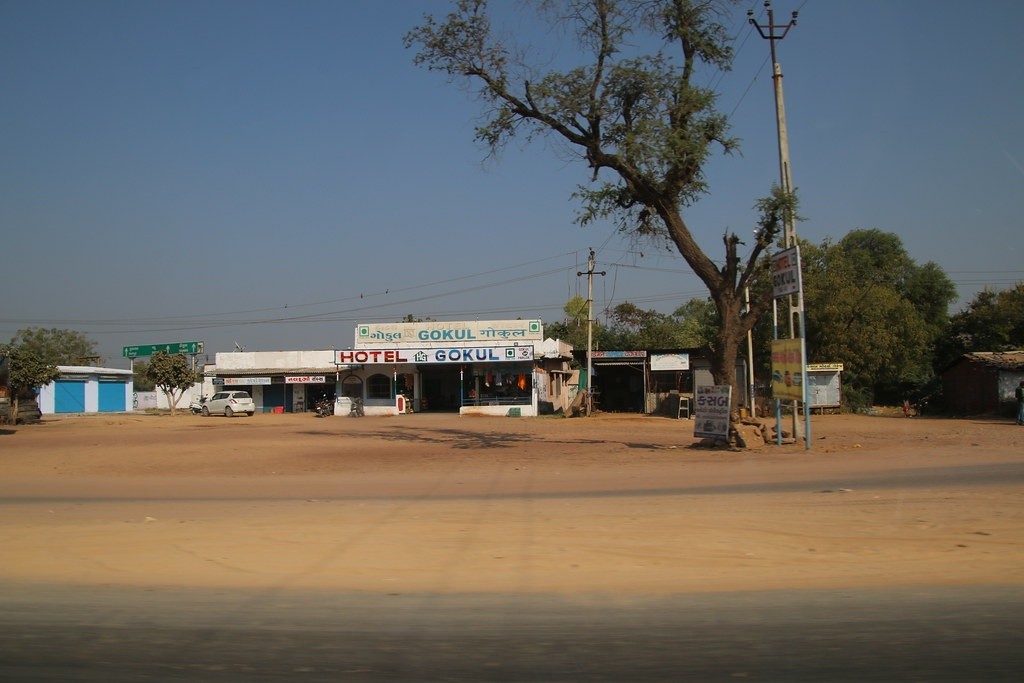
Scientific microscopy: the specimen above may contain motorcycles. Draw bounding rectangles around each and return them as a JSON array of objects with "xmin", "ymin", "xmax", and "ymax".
[
  {"xmin": 315, "ymin": 394, "xmax": 331, "ymax": 417},
  {"xmin": 350, "ymin": 399, "xmax": 365, "ymax": 417}
]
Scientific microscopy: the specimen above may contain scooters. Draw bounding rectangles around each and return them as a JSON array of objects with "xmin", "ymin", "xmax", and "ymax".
[{"xmin": 189, "ymin": 394, "xmax": 209, "ymax": 415}]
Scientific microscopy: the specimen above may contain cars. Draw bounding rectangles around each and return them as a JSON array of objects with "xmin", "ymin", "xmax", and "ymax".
[{"xmin": 202, "ymin": 391, "xmax": 255, "ymax": 417}]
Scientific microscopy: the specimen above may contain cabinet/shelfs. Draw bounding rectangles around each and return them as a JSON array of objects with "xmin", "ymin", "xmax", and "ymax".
[{"xmin": 668, "ymin": 393, "xmax": 690, "ymax": 420}]
